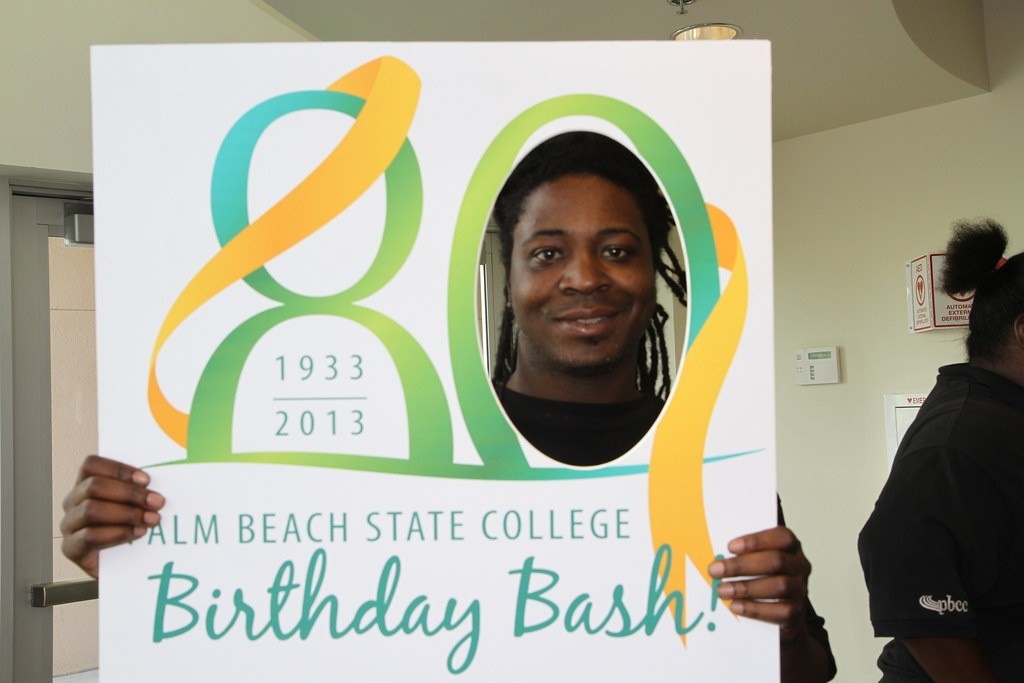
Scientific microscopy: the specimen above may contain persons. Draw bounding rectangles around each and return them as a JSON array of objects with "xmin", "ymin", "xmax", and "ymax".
[
  {"xmin": 857, "ymin": 217, "xmax": 1024, "ymax": 683},
  {"xmin": 61, "ymin": 131, "xmax": 838, "ymax": 683}
]
